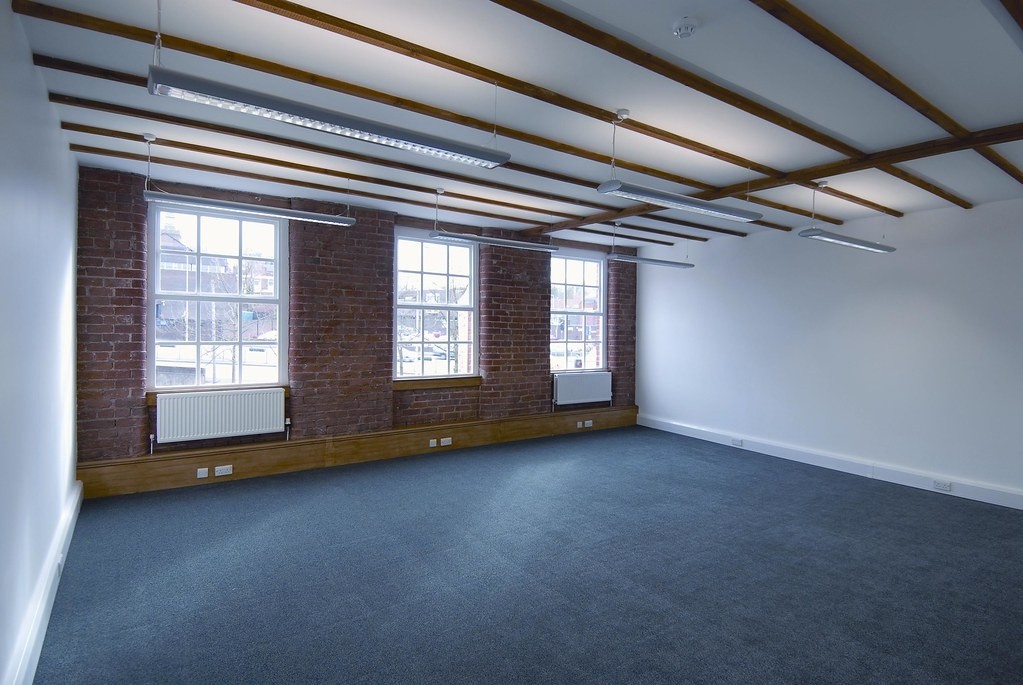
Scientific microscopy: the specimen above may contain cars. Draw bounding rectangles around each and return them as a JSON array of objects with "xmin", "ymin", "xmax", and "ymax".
[{"xmin": 397, "ymin": 332, "xmax": 455, "ymax": 362}]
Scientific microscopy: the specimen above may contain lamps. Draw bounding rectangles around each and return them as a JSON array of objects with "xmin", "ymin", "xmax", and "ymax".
[
  {"xmin": 607, "ymin": 253, "xmax": 694, "ymax": 270},
  {"xmin": 145, "ymin": 191, "xmax": 356, "ymax": 227},
  {"xmin": 431, "ymin": 230, "xmax": 558, "ymax": 253},
  {"xmin": 596, "ymin": 178, "xmax": 762, "ymax": 223},
  {"xmin": 799, "ymin": 229, "xmax": 895, "ymax": 253},
  {"xmin": 148, "ymin": 66, "xmax": 510, "ymax": 169}
]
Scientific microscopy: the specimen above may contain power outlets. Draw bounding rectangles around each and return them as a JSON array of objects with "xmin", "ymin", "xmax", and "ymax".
[
  {"xmin": 430, "ymin": 440, "xmax": 436, "ymax": 446},
  {"xmin": 215, "ymin": 465, "xmax": 232, "ymax": 476},
  {"xmin": 441, "ymin": 438, "xmax": 452, "ymax": 446},
  {"xmin": 197, "ymin": 468, "xmax": 208, "ymax": 478},
  {"xmin": 577, "ymin": 422, "xmax": 582, "ymax": 428},
  {"xmin": 585, "ymin": 420, "xmax": 593, "ymax": 427}
]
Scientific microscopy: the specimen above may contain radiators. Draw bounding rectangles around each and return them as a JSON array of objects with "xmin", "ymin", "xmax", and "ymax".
[
  {"xmin": 151, "ymin": 388, "xmax": 290, "ymax": 456},
  {"xmin": 552, "ymin": 372, "xmax": 612, "ymax": 412}
]
